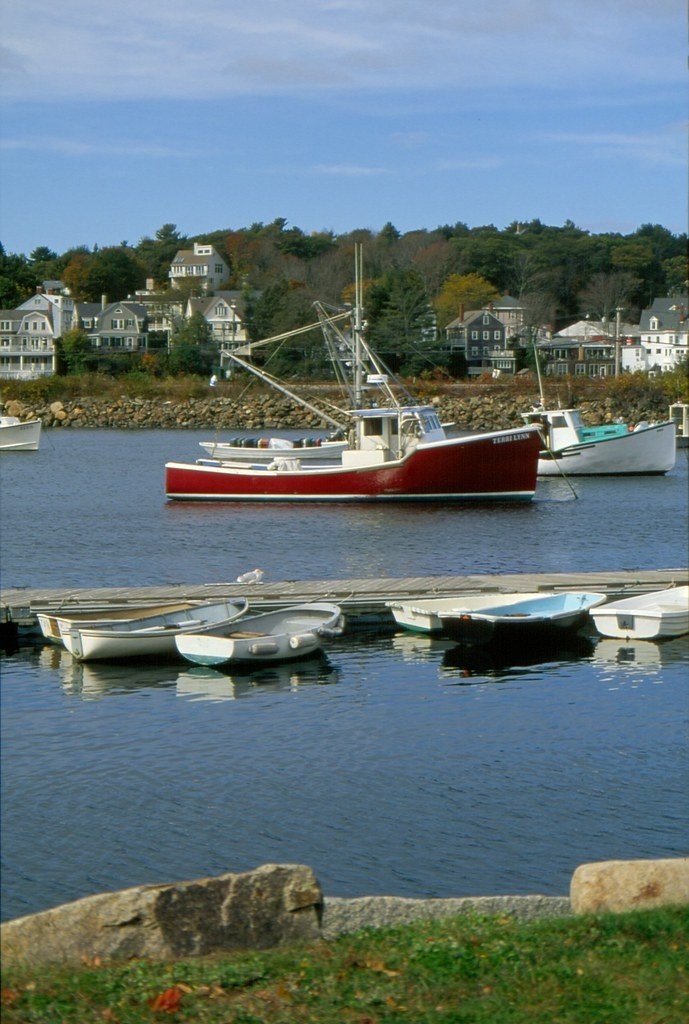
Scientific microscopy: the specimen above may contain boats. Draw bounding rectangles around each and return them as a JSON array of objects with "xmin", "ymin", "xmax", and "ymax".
[
  {"xmin": 520, "ymin": 335, "xmax": 678, "ymax": 476},
  {"xmin": 589, "ymin": 584, "xmax": 689, "ymax": 641},
  {"xmin": 437, "ymin": 588, "xmax": 607, "ymax": 648},
  {"xmin": 173, "ymin": 600, "xmax": 344, "ymax": 667},
  {"xmin": 667, "ymin": 399, "xmax": 689, "ymax": 448},
  {"xmin": 34, "ymin": 594, "xmax": 250, "ymax": 662},
  {"xmin": 385, "ymin": 590, "xmax": 554, "ymax": 635},
  {"xmin": 198, "ymin": 439, "xmax": 358, "ymax": 461},
  {"xmin": 0, "ymin": 415, "xmax": 43, "ymax": 452},
  {"xmin": 163, "ymin": 240, "xmax": 546, "ymax": 506}
]
